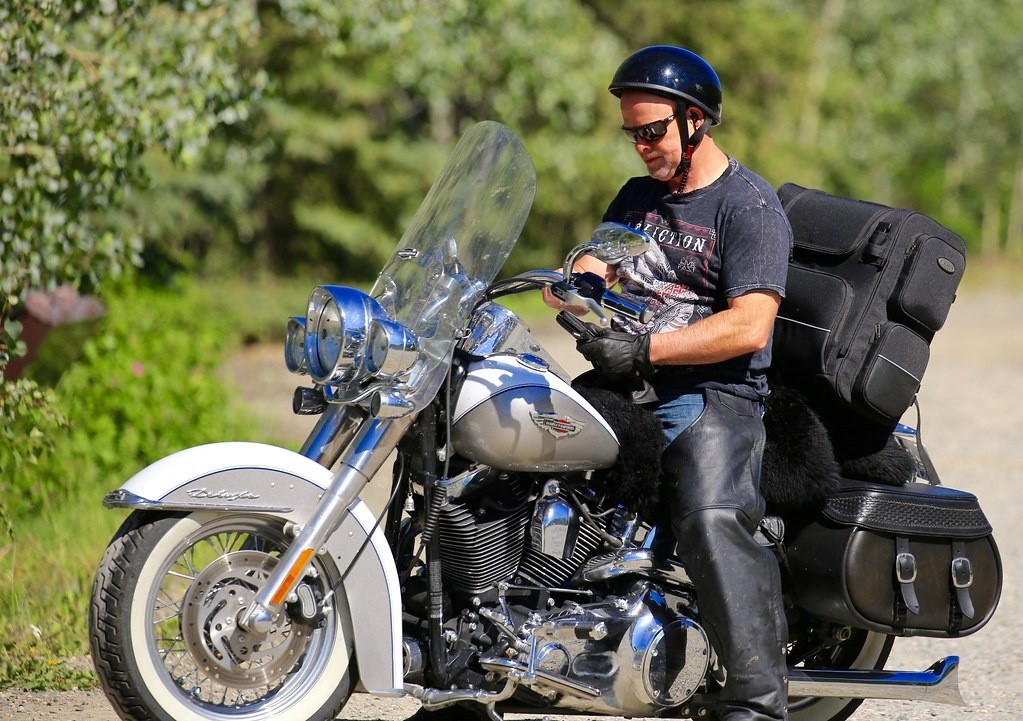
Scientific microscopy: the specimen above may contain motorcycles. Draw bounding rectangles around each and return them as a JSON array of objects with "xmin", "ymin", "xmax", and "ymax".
[{"xmin": 81, "ymin": 119, "xmax": 969, "ymax": 720}]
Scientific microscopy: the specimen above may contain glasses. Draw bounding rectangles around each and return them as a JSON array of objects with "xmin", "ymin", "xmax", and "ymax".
[{"xmin": 620, "ymin": 102, "xmax": 692, "ymax": 145}]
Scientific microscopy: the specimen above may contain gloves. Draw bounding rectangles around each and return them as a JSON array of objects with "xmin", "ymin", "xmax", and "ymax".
[{"xmin": 576, "ymin": 322, "xmax": 670, "ymax": 405}]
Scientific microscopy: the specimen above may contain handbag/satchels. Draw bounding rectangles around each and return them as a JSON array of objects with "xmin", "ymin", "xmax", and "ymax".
[{"xmin": 773, "ymin": 183, "xmax": 967, "ymax": 421}]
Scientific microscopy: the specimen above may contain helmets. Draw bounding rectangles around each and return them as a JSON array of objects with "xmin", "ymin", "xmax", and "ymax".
[{"xmin": 607, "ymin": 45, "xmax": 722, "ymax": 126}]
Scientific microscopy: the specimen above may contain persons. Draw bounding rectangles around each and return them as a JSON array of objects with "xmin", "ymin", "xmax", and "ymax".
[{"xmin": 401, "ymin": 46, "xmax": 790, "ymax": 720}]
